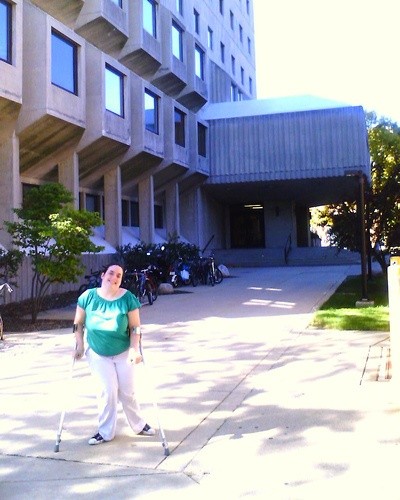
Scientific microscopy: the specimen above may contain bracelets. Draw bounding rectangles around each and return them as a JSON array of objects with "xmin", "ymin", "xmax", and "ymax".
[
  {"xmin": 128, "ymin": 327, "xmax": 140, "ymax": 336},
  {"xmin": 72, "ymin": 323, "xmax": 85, "ymax": 333}
]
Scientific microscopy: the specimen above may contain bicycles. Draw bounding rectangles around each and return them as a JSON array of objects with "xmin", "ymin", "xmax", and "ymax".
[{"xmin": 78, "ymin": 254, "xmax": 223, "ymax": 305}]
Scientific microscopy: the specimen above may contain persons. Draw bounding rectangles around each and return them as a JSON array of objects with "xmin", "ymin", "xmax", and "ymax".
[{"xmin": 71, "ymin": 264, "xmax": 156, "ymax": 444}]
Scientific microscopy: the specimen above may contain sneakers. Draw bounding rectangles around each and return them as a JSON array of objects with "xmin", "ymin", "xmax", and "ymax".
[
  {"xmin": 89, "ymin": 433, "xmax": 104, "ymax": 445},
  {"xmin": 142, "ymin": 424, "xmax": 156, "ymax": 435}
]
